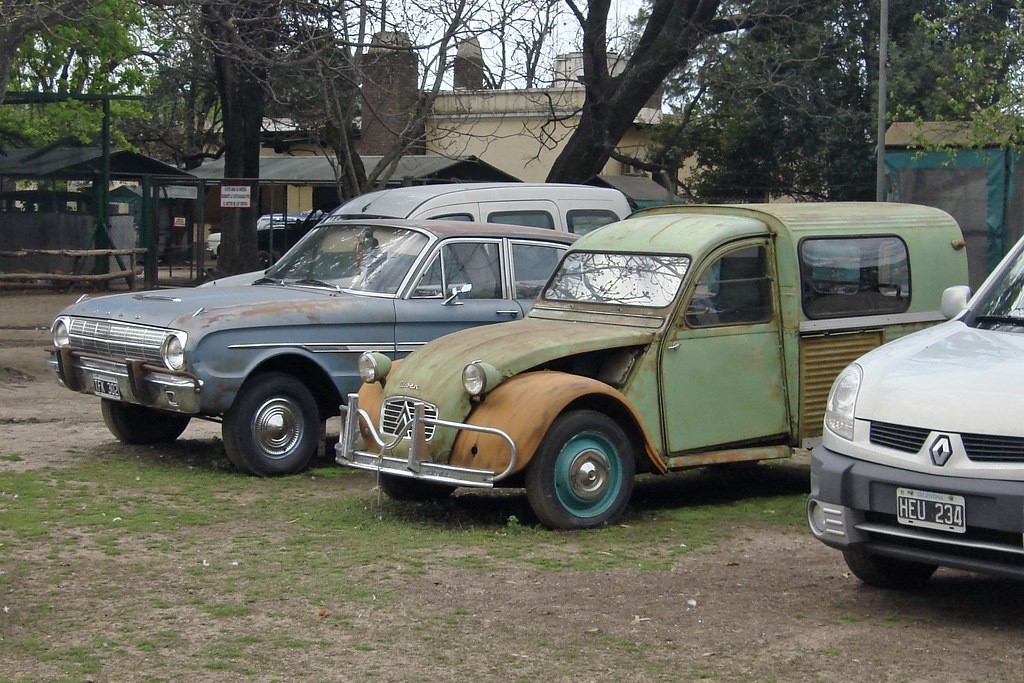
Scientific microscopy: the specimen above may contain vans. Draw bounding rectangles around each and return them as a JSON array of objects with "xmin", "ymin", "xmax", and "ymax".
[
  {"xmin": 804, "ymin": 233, "xmax": 1024, "ymax": 591},
  {"xmin": 329, "ymin": 181, "xmax": 631, "ymax": 238}
]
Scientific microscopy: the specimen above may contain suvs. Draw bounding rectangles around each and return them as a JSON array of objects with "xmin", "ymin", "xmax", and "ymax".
[{"xmin": 205, "ymin": 213, "xmax": 328, "ymax": 259}]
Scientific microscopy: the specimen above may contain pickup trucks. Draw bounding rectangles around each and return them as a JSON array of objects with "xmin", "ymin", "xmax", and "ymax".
[{"xmin": 333, "ymin": 205, "xmax": 968, "ymax": 525}]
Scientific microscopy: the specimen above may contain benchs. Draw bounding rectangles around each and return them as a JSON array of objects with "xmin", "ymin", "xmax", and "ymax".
[{"xmin": 805, "ymin": 280, "xmax": 909, "ymax": 317}]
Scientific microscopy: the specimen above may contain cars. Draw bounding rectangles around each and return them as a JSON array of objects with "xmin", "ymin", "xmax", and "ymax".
[{"xmin": 48, "ymin": 217, "xmax": 585, "ymax": 482}]
